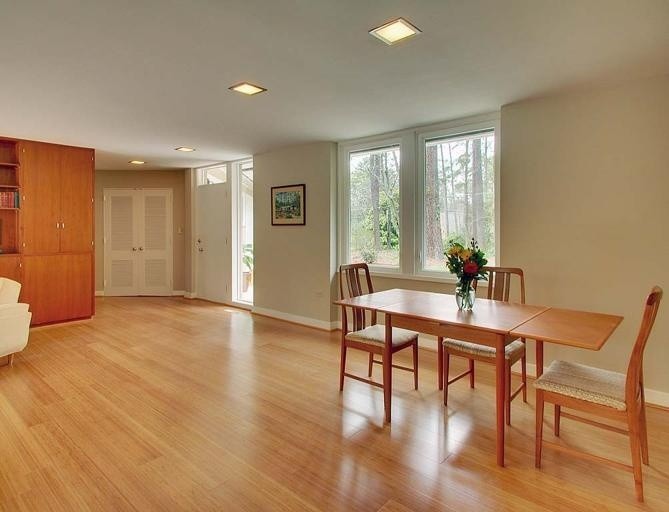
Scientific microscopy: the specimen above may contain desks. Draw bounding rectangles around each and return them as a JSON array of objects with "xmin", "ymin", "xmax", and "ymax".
[{"xmin": 333, "ymin": 288, "xmax": 625, "ymax": 468}]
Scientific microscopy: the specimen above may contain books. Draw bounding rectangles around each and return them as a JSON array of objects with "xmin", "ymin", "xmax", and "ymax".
[{"xmin": 0, "ymin": 191, "xmax": 19, "ymax": 208}]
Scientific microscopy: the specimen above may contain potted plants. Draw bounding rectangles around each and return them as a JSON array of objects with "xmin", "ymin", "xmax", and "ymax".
[{"xmin": 243, "ymin": 243, "xmax": 253, "ymax": 292}]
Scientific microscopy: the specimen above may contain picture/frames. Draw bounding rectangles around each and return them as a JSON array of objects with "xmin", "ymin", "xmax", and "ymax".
[{"xmin": 271, "ymin": 183, "xmax": 306, "ymax": 226}]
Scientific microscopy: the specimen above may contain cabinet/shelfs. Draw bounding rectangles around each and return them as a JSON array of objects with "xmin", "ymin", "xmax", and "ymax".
[{"xmin": 0, "ymin": 136, "xmax": 95, "ymax": 328}]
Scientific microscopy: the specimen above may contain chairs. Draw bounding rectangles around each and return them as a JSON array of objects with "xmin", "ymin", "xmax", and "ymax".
[
  {"xmin": 339, "ymin": 263, "xmax": 419, "ymax": 408},
  {"xmin": 532, "ymin": 287, "xmax": 663, "ymax": 504},
  {"xmin": 443, "ymin": 267, "xmax": 527, "ymax": 425},
  {"xmin": 0, "ymin": 277, "xmax": 32, "ymax": 366}
]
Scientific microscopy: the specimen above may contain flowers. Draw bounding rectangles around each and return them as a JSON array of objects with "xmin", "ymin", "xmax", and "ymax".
[{"xmin": 444, "ymin": 234, "xmax": 488, "ymax": 311}]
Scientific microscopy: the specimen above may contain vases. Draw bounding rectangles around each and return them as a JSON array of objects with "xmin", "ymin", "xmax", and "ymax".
[{"xmin": 453, "ymin": 285, "xmax": 476, "ymax": 311}]
